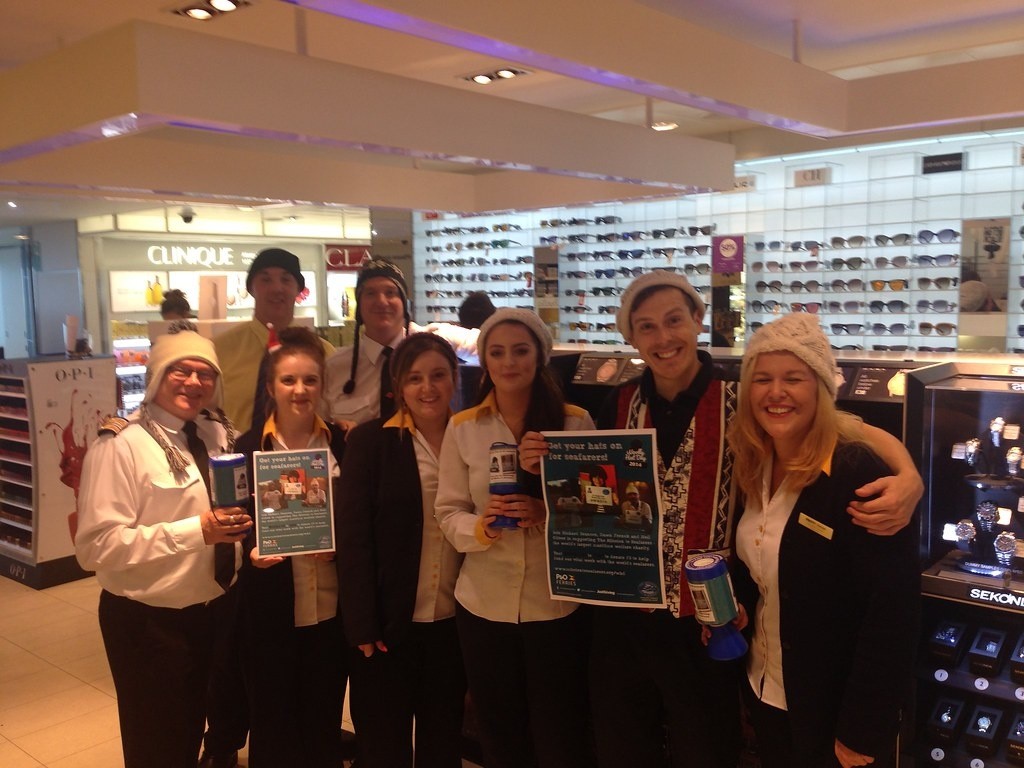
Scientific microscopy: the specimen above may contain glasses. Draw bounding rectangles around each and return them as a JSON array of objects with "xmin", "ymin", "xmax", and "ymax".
[
  {"xmin": 420, "ymin": 214, "xmax": 1024, "ymax": 363},
  {"xmin": 169, "ymin": 365, "xmax": 215, "ymax": 386}
]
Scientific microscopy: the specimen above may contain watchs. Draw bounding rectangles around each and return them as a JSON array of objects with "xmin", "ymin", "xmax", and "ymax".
[{"xmin": 935, "ymin": 416, "xmax": 1024, "ymax": 737}]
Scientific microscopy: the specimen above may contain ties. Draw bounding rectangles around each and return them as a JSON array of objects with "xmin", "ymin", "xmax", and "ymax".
[
  {"xmin": 379, "ymin": 346, "xmax": 395, "ymax": 423},
  {"xmin": 181, "ymin": 420, "xmax": 236, "ymax": 592}
]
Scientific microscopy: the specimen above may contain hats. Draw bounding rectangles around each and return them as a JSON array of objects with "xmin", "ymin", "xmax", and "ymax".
[
  {"xmin": 616, "ymin": 270, "xmax": 704, "ymax": 345},
  {"xmin": 140, "ymin": 319, "xmax": 234, "ymax": 485},
  {"xmin": 739, "ymin": 312, "xmax": 838, "ymax": 403},
  {"xmin": 246, "ymin": 248, "xmax": 305, "ymax": 298},
  {"xmin": 459, "ymin": 292, "xmax": 497, "ymax": 329},
  {"xmin": 342, "ymin": 256, "xmax": 410, "ymax": 394},
  {"xmin": 477, "ymin": 307, "xmax": 553, "ymax": 371}
]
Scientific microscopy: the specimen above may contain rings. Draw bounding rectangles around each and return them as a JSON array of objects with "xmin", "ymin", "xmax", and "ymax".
[{"xmin": 229, "ymin": 515, "xmax": 235, "ymax": 525}]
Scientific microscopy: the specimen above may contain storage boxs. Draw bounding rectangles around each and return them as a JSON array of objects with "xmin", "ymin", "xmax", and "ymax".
[{"xmin": 925, "ymin": 619, "xmax": 1024, "ymax": 765}]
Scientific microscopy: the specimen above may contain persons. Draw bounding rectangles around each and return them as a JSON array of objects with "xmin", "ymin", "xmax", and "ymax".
[
  {"xmin": 489, "ymin": 457, "xmax": 499, "ymax": 472},
  {"xmin": 158, "ymin": 287, "xmax": 200, "ymax": 320},
  {"xmin": 626, "ymin": 441, "xmax": 646, "ymax": 466},
  {"xmin": 303, "ymin": 479, "xmax": 327, "ymax": 508},
  {"xmin": 282, "ymin": 470, "xmax": 306, "ymax": 500},
  {"xmin": 454, "ymin": 294, "xmax": 501, "ymax": 407},
  {"xmin": 696, "ymin": 314, "xmax": 921, "ymax": 768},
  {"xmin": 311, "ymin": 454, "xmax": 325, "ymax": 470},
  {"xmin": 324, "ymin": 261, "xmax": 483, "ymax": 421},
  {"xmin": 210, "ymin": 248, "xmax": 339, "ymax": 434},
  {"xmin": 262, "ymin": 482, "xmax": 283, "ymax": 509},
  {"xmin": 515, "ymin": 271, "xmax": 924, "ymax": 768},
  {"xmin": 694, "ymin": 589, "xmax": 708, "ymax": 609},
  {"xmin": 584, "ymin": 466, "xmax": 623, "ymax": 515},
  {"xmin": 556, "ymin": 480, "xmax": 583, "ymax": 527},
  {"xmin": 73, "ymin": 307, "xmax": 611, "ymax": 768},
  {"xmin": 620, "ymin": 485, "xmax": 653, "ymax": 532},
  {"xmin": 236, "ymin": 474, "xmax": 246, "ymax": 491}
]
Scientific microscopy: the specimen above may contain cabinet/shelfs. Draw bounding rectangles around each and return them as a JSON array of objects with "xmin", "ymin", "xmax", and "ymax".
[
  {"xmin": 0, "ymin": 377, "xmax": 33, "ymax": 558},
  {"xmin": 904, "ymin": 545, "xmax": 1024, "ymax": 768},
  {"xmin": 421, "ymin": 162, "xmax": 1024, "ymax": 339}
]
